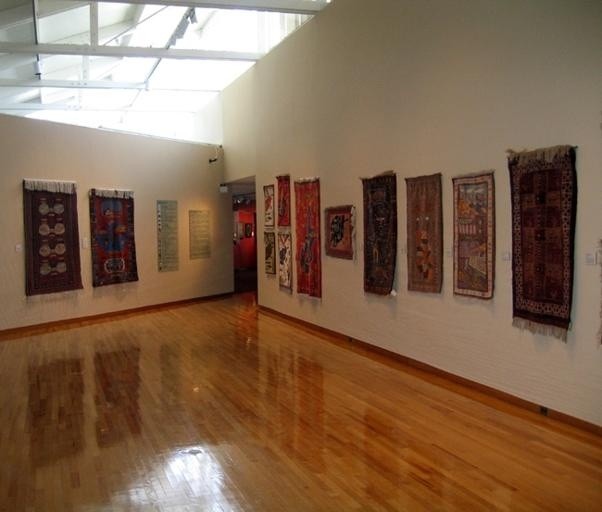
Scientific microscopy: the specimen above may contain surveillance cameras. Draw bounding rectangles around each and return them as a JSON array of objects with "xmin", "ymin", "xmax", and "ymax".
[{"xmin": 216, "ymin": 144, "xmax": 223, "ymax": 152}]
